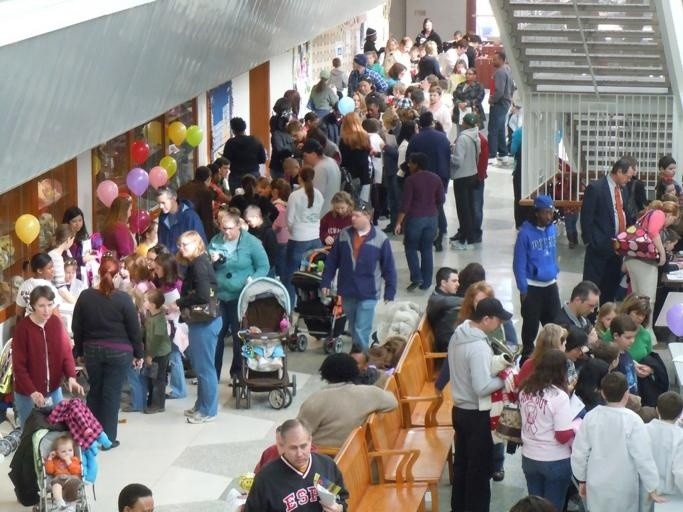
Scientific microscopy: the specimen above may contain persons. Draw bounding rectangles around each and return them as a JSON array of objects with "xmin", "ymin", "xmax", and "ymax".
[
  {"xmin": 166, "ymin": 230, "xmax": 223, "ymax": 424},
  {"xmin": 71, "ymin": 259, "xmax": 144, "ymax": 450},
  {"xmin": 426, "ymin": 153, "xmax": 683, "ymax": 511},
  {"xmin": 267, "ymin": 15, "xmax": 523, "ymax": 252},
  {"xmin": 44, "ymin": 436, "xmax": 82, "ymax": 510},
  {"xmin": 246, "ymin": 419, "xmax": 349, "ymax": 512},
  {"xmin": 296, "ymin": 352, "xmax": 398, "ymax": 453},
  {"xmin": 14, "ymin": 117, "xmax": 452, "ymax": 412},
  {"xmin": 12, "ymin": 286, "xmax": 86, "ymax": 431},
  {"xmin": 253, "ymin": 427, "xmax": 318, "ymax": 476},
  {"xmin": 118, "ymin": 484, "xmax": 154, "ymax": 512}
]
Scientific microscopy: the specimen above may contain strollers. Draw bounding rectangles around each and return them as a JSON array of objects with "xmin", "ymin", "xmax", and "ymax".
[
  {"xmin": 28, "ymin": 410, "xmax": 89, "ymax": 512},
  {"xmin": 229, "ymin": 278, "xmax": 297, "ymax": 409},
  {"xmin": 290, "ymin": 247, "xmax": 347, "ymax": 355}
]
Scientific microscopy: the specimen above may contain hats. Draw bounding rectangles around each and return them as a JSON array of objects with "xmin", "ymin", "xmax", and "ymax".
[
  {"xmin": 365, "ymin": 27, "xmax": 377, "ymax": 36},
  {"xmin": 463, "ymin": 113, "xmax": 477, "ymax": 127},
  {"xmin": 532, "ymin": 193, "xmax": 555, "ymax": 209},
  {"xmin": 281, "ymin": 157, "xmax": 299, "ymax": 172},
  {"xmin": 319, "ymin": 69, "xmax": 332, "ymax": 79},
  {"xmin": 474, "ymin": 296, "xmax": 513, "ymax": 321}
]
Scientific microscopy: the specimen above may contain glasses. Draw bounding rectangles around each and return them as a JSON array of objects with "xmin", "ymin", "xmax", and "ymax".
[
  {"xmin": 158, "ymin": 184, "xmax": 171, "ymax": 191},
  {"xmin": 65, "ymin": 259, "xmax": 77, "ymax": 265},
  {"xmin": 636, "ymin": 293, "xmax": 651, "ymax": 301}
]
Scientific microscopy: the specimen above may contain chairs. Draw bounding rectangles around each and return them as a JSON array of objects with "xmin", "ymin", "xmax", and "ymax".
[
  {"xmin": 391, "ymin": 329, "xmax": 456, "ymax": 485},
  {"xmin": 367, "ymin": 374, "xmax": 454, "ymax": 512},
  {"xmin": 331, "ymin": 427, "xmax": 428, "ymax": 512},
  {"xmin": 418, "ymin": 313, "xmax": 450, "ymax": 382}
]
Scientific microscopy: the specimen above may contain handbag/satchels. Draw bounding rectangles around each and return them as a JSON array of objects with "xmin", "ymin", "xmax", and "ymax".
[
  {"xmin": 180, "ymin": 288, "xmax": 217, "ymax": 323},
  {"xmin": 609, "ymin": 221, "xmax": 660, "ymax": 260}
]
